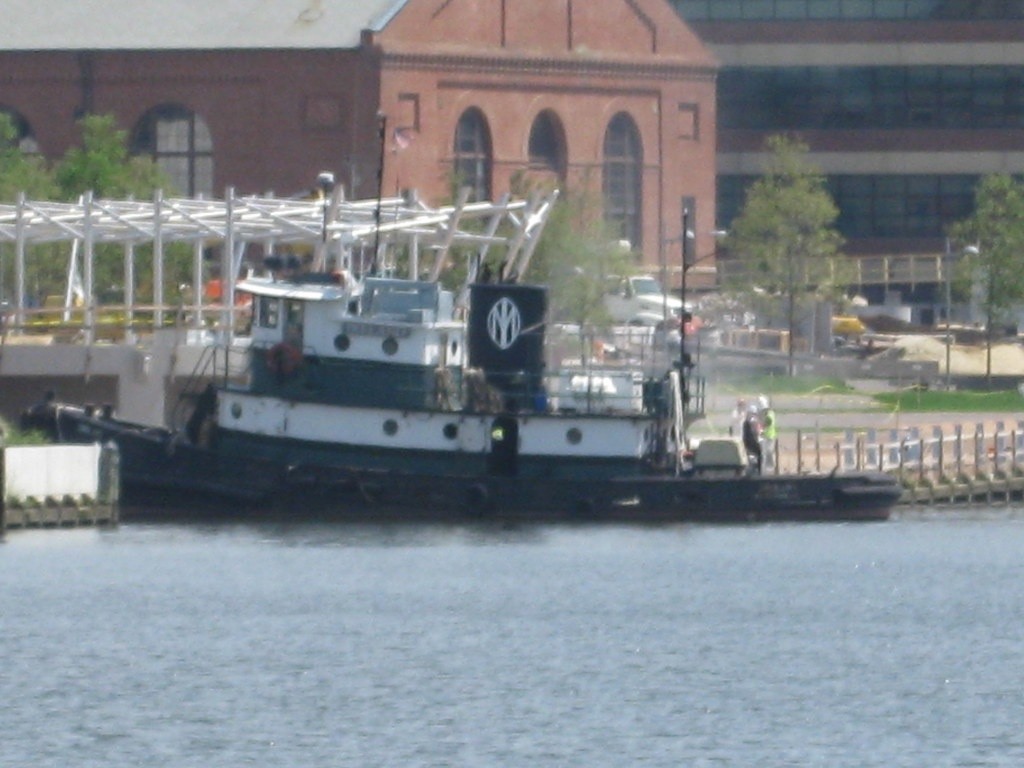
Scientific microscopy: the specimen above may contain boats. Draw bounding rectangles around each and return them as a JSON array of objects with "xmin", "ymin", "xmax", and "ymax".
[{"xmin": 16, "ymin": 270, "xmax": 902, "ymax": 522}]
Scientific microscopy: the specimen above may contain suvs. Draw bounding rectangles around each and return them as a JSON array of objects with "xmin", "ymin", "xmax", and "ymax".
[{"xmin": 602, "ymin": 275, "xmax": 701, "ymax": 325}]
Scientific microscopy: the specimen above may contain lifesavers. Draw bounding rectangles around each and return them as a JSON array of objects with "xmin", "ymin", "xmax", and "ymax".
[{"xmin": 267, "ymin": 342, "xmax": 303, "ymax": 372}]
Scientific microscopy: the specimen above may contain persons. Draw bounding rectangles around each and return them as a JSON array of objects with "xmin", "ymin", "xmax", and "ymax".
[
  {"xmin": 728, "ymin": 398, "xmax": 747, "ymax": 438},
  {"xmin": 743, "ymin": 405, "xmax": 765, "ymax": 474},
  {"xmin": 755, "ymin": 394, "xmax": 776, "ymax": 468}
]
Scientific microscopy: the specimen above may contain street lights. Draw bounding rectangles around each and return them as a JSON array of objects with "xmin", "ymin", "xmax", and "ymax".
[{"xmin": 317, "ymin": 171, "xmax": 334, "ymax": 278}]
